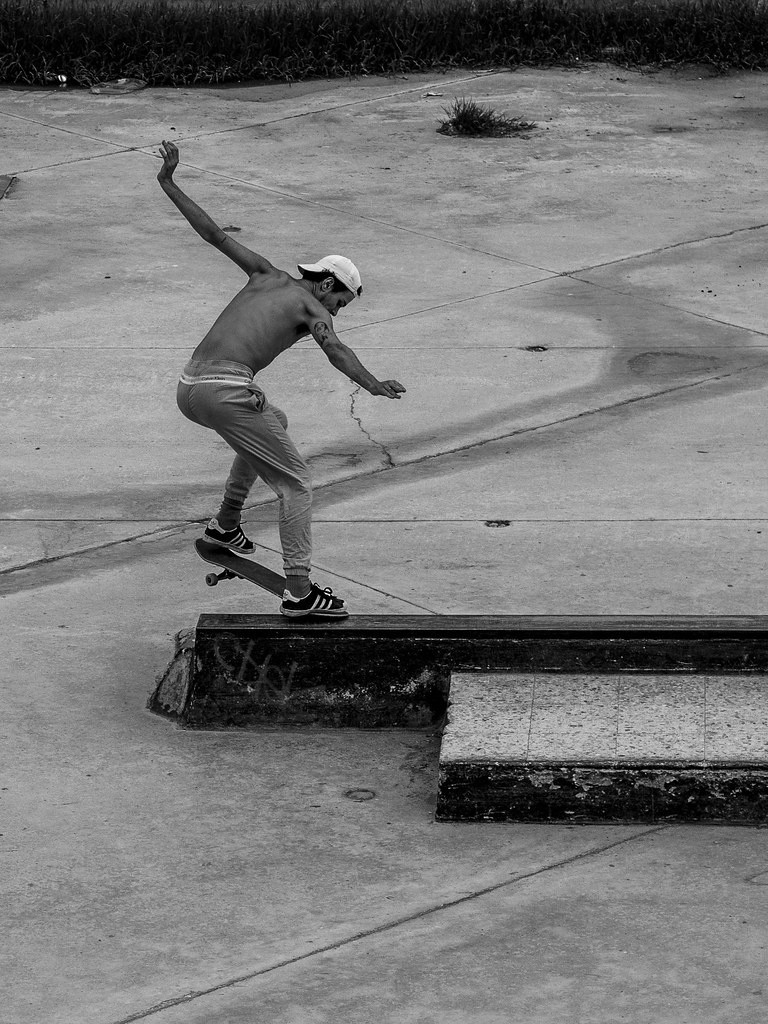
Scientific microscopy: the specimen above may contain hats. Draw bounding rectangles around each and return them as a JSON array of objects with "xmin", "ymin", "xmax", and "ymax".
[{"xmin": 297, "ymin": 256, "xmax": 363, "ymax": 300}]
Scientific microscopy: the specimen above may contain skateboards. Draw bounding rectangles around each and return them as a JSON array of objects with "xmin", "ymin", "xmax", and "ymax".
[{"xmin": 194, "ymin": 538, "xmax": 350, "ymax": 617}]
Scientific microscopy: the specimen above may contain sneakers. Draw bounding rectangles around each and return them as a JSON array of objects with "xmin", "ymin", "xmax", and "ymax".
[
  {"xmin": 203, "ymin": 515, "xmax": 256, "ymax": 554},
  {"xmin": 279, "ymin": 584, "xmax": 348, "ymax": 617}
]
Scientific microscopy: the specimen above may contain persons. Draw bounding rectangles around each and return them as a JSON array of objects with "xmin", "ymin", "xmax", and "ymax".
[{"xmin": 156, "ymin": 140, "xmax": 407, "ymax": 614}]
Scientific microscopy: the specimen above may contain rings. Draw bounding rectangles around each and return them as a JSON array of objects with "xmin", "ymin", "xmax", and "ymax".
[{"xmin": 388, "ymin": 388, "xmax": 391, "ymax": 390}]
